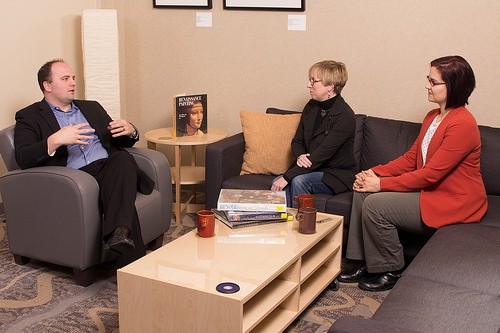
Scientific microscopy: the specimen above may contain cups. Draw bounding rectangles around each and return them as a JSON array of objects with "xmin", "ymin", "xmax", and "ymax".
[
  {"xmin": 197, "ymin": 210, "xmax": 215, "ymax": 237},
  {"xmin": 292, "ymin": 194, "xmax": 314, "ymax": 212},
  {"xmin": 296, "ymin": 207, "xmax": 317, "ymax": 234}
]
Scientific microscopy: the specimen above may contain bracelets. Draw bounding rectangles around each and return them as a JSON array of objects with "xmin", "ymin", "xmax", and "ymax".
[{"xmin": 297, "ymin": 156, "xmax": 300, "ymax": 159}]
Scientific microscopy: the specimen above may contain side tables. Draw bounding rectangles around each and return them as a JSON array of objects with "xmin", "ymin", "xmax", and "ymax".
[{"xmin": 145, "ymin": 126, "xmax": 231, "ymax": 226}]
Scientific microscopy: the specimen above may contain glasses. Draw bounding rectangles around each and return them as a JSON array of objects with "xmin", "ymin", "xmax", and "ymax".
[
  {"xmin": 309, "ymin": 77, "xmax": 321, "ymax": 86},
  {"xmin": 427, "ymin": 76, "xmax": 448, "ymax": 87}
]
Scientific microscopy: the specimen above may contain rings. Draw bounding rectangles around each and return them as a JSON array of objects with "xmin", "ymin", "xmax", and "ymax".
[
  {"xmin": 354, "ymin": 181, "xmax": 357, "ymax": 184},
  {"xmin": 360, "ymin": 185, "xmax": 363, "ymax": 189},
  {"xmin": 122, "ymin": 126, "xmax": 125, "ymax": 131}
]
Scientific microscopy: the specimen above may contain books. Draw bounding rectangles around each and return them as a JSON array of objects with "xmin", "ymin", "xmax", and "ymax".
[
  {"xmin": 211, "ymin": 189, "xmax": 294, "ymax": 230},
  {"xmin": 173, "ymin": 92, "xmax": 207, "ymax": 137}
]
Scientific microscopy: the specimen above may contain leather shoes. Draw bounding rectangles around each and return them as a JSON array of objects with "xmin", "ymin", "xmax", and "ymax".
[
  {"xmin": 104, "ymin": 234, "xmax": 135, "ymax": 257},
  {"xmin": 358, "ymin": 271, "xmax": 401, "ymax": 291},
  {"xmin": 113, "ymin": 254, "xmax": 146, "ymax": 271},
  {"xmin": 338, "ymin": 264, "xmax": 370, "ymax": 283}
]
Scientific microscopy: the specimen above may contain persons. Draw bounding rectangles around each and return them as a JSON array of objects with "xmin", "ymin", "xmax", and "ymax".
[
  {"xmin": 13, "ymin": 58, "xmax": 156, "ymax": 275},
  {"xmin": 271, "ymin": 60, "xmax": 360, "ymax": 208},
  {"xmin": 184, "ymin": 100, "xmax": 205, "ymax": 136},
  {"xmin": 335, "ymin": 56, "xmax": 488, "ymax": 292}
]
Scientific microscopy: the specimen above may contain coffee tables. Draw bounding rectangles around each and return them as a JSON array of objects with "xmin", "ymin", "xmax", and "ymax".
[{"xmin": 117, "ymin": 206, "xmax": 344, "ymax": 333}]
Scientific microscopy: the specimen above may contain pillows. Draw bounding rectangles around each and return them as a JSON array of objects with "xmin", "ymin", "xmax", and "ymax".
[{"xmin": 237, "ymin": 110, "xmax": 301, "ymax": 176}]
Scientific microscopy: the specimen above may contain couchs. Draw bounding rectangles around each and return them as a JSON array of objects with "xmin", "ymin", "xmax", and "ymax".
[
  {"xmin": 204, "ymin": 108, "xmax": 500, "ymax": 262},
  {"xmin": 328, "ymin": 221, "xmax": 500, "ymax": 333},
  {"xmin": 0, "ymin": 122, "xmax": 173, "ymax": 287}
]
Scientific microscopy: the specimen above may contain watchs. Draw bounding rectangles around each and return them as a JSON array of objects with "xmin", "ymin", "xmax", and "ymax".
[{"xmin": 129, "ymin": 129, "xmax": 137, "ymax": 139}]
